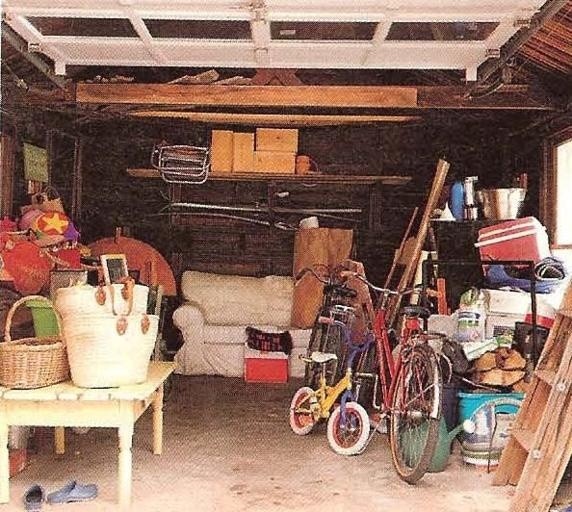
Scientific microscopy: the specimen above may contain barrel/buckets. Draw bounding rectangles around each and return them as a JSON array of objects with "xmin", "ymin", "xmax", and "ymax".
[
  {"xmin": 456, "ymin": 391, "xmax": 524, "ymax": 465},
  {"xmin": 489, "ymin": 188, "xmax": 527, "ymax": 220},
  {"xmin": 477, "ymin": 188, "xmax": 496, "ymax": 221},
  {"xmin": 456, "ymin": 305, "xmax": 486, "ymax": 342}
]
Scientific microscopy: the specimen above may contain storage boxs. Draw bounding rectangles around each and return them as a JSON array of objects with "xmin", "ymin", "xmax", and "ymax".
[
  {"xmin": 209, "ymin": 129, "xmax": 299, "ymax": 174},
  {"xmin": 472, "ymin": 217, "xmax": 558, "ymax": 338}
]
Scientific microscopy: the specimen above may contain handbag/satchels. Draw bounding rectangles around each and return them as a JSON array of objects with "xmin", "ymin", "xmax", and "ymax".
[{"xmin": 57, "ymin": 254, "xmax": 160, "ymax": 389}]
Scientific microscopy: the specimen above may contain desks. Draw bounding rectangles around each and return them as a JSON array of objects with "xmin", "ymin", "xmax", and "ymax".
[{"xmin": 0, "ymin": 360, "xmax": 177, "ymax": 509}]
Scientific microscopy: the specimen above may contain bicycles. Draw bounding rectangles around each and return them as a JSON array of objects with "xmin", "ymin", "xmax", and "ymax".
[
  {"xmin": 292, "ymin": 261, "xmax": 357, "ymax": 388},
  {"xmin": 288, "ymin": 349, "xmax": 370, "ymax": 456},
  {"xmin": 336, "ymin": 268, "xmax": 446, "ymax": 486}
]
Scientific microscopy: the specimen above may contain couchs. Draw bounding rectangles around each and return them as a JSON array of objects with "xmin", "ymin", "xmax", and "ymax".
[{"xmin": 172, "ymin": 271, "xmax": 318, "ymax": 376}]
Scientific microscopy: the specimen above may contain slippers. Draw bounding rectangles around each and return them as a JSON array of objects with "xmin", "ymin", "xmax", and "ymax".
[
  {"xmin": 24, "ymin": 483, "xmax": 45, "ymax": 512},
  {"xmin": 47, "ymin": 482, "xmax": 98, "ymax": 504}
]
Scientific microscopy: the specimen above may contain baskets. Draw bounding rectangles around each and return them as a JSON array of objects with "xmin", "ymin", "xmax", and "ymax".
[{"xmin": 0, "ymin": 295, "xmax": 70, "ymax": 390}]
